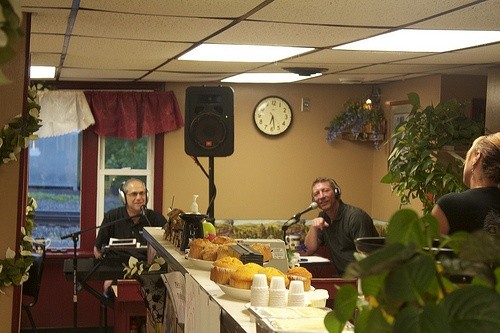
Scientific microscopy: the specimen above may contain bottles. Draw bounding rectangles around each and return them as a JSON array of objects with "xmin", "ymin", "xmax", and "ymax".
[{"xmin": 202, "ymin": 220, "xmax": 216, "ymax": 237}]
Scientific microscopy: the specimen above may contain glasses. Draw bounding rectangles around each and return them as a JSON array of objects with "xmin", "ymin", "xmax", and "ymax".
[{"xmin": 127, "ymin": 190, "xmax": 146, "ymax": 197}]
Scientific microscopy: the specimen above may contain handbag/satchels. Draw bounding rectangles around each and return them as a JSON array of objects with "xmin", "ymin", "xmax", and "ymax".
[{"xmin": 101, "ymin": 246, "xmax": 148, "ymax": 267}]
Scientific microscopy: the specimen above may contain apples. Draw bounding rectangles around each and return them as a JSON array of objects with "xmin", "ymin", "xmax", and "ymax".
[{"xmin": 202, "ymin": 222, "xmax": 216, "ymax": 237}]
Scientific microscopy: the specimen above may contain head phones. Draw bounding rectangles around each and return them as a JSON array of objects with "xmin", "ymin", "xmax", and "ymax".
[
  {"xmin": 118, "ymin": 181, "xmax": 149, "ymax": 205},
  {"xmin": 312, "ymin": 179, "xmax": 341, "ymax": 203}
]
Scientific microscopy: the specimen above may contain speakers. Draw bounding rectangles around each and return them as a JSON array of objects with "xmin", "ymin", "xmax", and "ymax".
[{"xmin": 184, "ymin": 85, "xmax": 234, "ymax": 157}]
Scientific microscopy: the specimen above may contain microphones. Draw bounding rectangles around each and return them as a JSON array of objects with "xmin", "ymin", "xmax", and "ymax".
[
  {"xmin": 140, "ymin": 205, "xmax": 147, "ymax": 216},
  {"xmin": 292, "ymin": 202, "xmax": 318, "ymax": 218}
]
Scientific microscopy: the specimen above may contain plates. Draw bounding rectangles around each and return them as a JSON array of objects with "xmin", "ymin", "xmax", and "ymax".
[
  {"xmin": 353, "ymin": 237, "xmax": 458, "ymax": 262},
  {"xmin": 218, "ymin": 283, "xmax": 316, "ymax": 302},
  {"xmin": 186, "ymin": 252, "xmax": 215, "ymax": 270}
]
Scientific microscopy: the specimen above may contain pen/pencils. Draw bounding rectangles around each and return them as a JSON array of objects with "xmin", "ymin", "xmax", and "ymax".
[{"xmin": 301, "ymin": 259, "xmax": 308, "ymax": 261}]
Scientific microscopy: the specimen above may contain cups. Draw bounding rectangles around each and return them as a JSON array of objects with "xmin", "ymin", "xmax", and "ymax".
[
  {"xmin": 250, "ymin": 274, "xmax": 306, "ymax": 307},
  {"xmin": 285, "ymin": 235, "xmax": 300, "ymax": 249},
  {"xmin": 34, "ymin": 238, "xmax": 51, "ymax": 254}
]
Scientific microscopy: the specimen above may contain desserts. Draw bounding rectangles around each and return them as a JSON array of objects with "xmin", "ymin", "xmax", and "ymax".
[
  {"xmin": 210, "ymin": 256, "xmax": 312, "ymax": 290},
  {"xmin": 188, "ymin": 234, "xmax": 273, "ymax": 261}
]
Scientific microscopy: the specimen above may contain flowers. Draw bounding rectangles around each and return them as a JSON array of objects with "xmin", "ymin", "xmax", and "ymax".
[
  {"xmin": 378, "ymin": 91, "xmax": 484, "ymax": 217},
  {"xmin": 324, "ymin": 95, "xmax": 389, "ymax": 151}
]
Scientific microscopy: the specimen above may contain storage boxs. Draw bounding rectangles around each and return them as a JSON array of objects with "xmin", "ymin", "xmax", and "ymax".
[{"xmin": 227, "ymin": 242, "xmax": 264, "ymax": 267}]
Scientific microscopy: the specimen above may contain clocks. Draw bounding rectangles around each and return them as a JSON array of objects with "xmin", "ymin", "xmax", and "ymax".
[{"xmin": 253, "ymin": 95, "xmax": 293, "ymax": 138}]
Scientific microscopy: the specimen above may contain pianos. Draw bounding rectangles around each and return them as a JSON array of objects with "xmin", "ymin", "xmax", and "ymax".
[{"xmin": 63, "ymin": 257, "xmax": 148, "ymax": 333}]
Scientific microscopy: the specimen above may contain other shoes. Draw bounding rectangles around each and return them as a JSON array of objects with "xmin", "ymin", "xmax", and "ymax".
[{"xmin": 104, "ymin": 292, "xmax": 114, "ymax": 303}]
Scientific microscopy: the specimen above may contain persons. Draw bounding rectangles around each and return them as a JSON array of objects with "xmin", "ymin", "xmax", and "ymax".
[
  {"xmin": 424, "ymin": 131, "xmax": 500, "ymax": 283},
  {"xmin": 94, "ymin": 179, "xmax": 168, "ymax": 297},
  {"xmin": 304, "ymin": 176, "xmax": 379, "ymax": 279}
]
